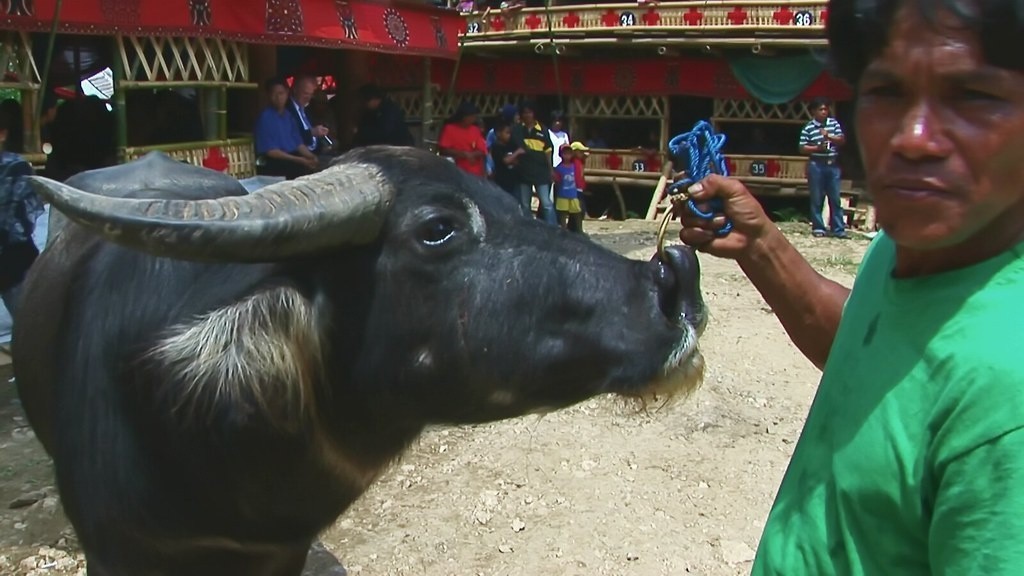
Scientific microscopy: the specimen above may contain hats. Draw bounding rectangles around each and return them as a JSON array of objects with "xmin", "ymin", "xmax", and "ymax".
[
  {"xmin": 570, "ymin": 141, "xmax": 589, "ymax": 151},
  {"xmin": 559, "ymin": 144, "xmax": 572, "ymax": 154}
]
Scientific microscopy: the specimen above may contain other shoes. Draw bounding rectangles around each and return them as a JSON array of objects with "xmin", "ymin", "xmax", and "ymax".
[
  {"xmin": 840, "ymin": 235, "xmax": 852, "ymax": 239},
  {"xmin": 813, "ymin": 232, "xmax": 824, "ymax": 237}
]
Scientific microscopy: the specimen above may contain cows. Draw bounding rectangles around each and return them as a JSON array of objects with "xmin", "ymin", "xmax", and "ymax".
[{"xmin": 12, "ymin": 148, "xmax": 709, "ymax": 576}]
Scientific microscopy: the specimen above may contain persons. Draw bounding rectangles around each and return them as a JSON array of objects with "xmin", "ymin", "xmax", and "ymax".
[
  {"xmin": 0, "ymin": 94, "xmax": 58, "ymax": 322},
  {"xmin": 677, "ymin": 0, "xmax": 1024, "ymax": 575},
  {"xmin": 798, "ymin": 97, "xmax": 853, "ymax": 239},
  {"xmin": 254, "ymin": 70, "xmax": 591, "ymax": 234}
]
